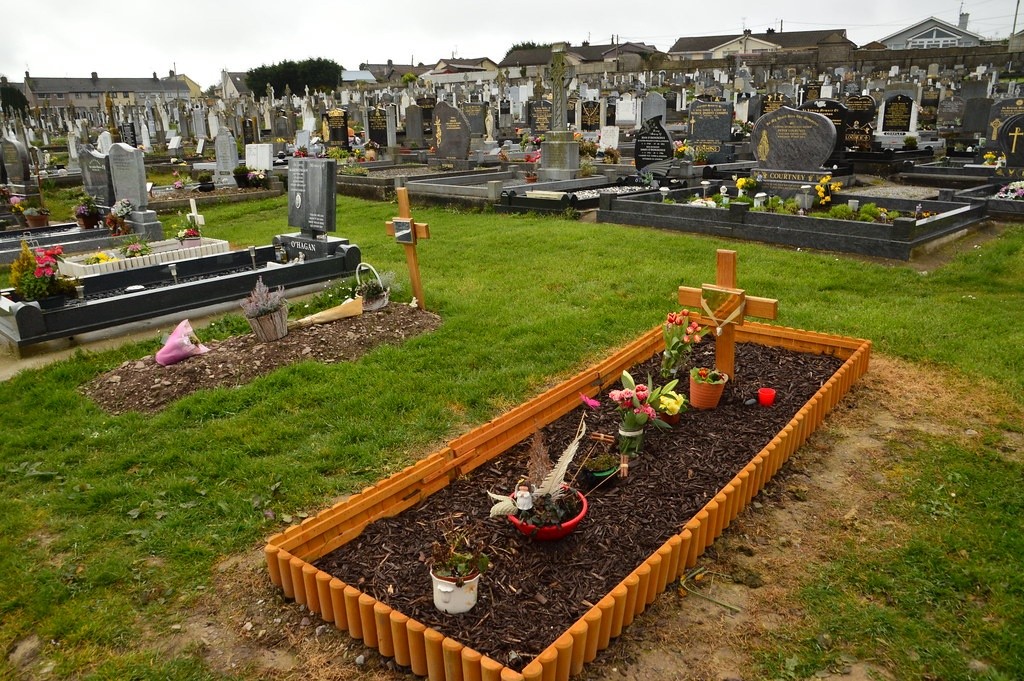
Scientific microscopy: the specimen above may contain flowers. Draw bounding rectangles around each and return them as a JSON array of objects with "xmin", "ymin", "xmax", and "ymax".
[
  {"xmin": 275, "ymin": 130, "xmax": 1024, "ymax": 205},
  {"xmin": 10, "ymin": 195, "xmax": 41, "ymax": 214},
  {"xmin": 577, "ymin": 370, "xmax": 679, "ymax": 457},
  {"xmin": 690, "ymin": 367, "xmax": 729, "ymax": 385},
  {"xmin": 172, "ymin": 171, "xmax": 192, "ymax": 190},
  {"xmin": 652, "ymin": 391, "xmax": 689, "ymax": 415},
  {"xmin": 21, "ymin": 245, "xmax": 64, "ymax": 301},
  {"xmin": 105, "ymin": 198, "xmax": 133, "ymax": 236},
  {"xmin": 119, "ymin": 230, "xmax": 152, "ymax": 258},
  {"xmin": 83, "ymin": 252, "xmax": 118, "ymax": 265},
  {"xmin": 169, "ymin": 210, "xmax": 203, "ymax": 245},
  {"xmin": 247, "ymin": 168, "xmax": 267, "ymax": 187},
  {"xmin": 71, "ymin": 193, "xmax": 98, "ymax": 217},
  {"xmin": 660, "ymin": 309, "xmax": 711, "ymax": 379}
]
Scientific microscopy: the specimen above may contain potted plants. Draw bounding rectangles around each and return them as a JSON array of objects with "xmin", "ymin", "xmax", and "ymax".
[
  {"xmin": 486, "ymin": 418, "xmax": 587, "ymax": 542},
  {"xmin": 902, "ymin": 136, "xmax": 918, "ymax": 152},
  {"xmin": 197, "ymin": 173, "xmax": 216, "ymax": 193},
  {"xmin": 23, "ymin": 206, "xmax": 50, "ymax": 228},
  {"xmin": 418, "ymin": 515, "xmax": 488, "ymax": 615},
  {"xmin": 232, "ymin": 165, "xmax": 249, "ymax": 189},
  {"xmin": 0, "ymin": 219, "xmax": 7, "ymax": 231},
  {"xmin": 525, "ymin": 168, "xmax": 539, "ymax": 184},
  {"xmin": 240, "ymin": 275, "xmax": 288, "ymax": 342},
  {"xmin": 52, "ymin": 155, "xmax": 70, "ymax": 170}
]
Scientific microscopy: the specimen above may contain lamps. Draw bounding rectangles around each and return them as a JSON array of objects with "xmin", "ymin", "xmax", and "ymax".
[
  {"xmin": 754, "ymin": 192, "xmax": 767, "ymax": 206},
  {"xmin": 700, "ymin": 181, "xmax": 711, "ymax": 198},
  {"xmin": 801, "ymin": 185, "xmax": 811, "ymax": 209},
  {"xmin": 248, "ymin": 245, "xmax": 257, "ymax": 270},
  {"xmin": 75, "ymin": 285, "xmax": 84, "ymax": 303},
  {"xmin": 659, "ymin": 187, "xmax": 670, "ymax": 202},
  {"xmin": 168, "ymin": 264, "xmax": 178, "ymax": 285}
]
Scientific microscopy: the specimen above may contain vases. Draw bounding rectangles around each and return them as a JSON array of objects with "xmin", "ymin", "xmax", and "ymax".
[
  {"xmin": 660, "ymin": 413, "xmax": 681, "ymax": 424},
  {"xmin": 689, "ymin": 376, "xmax": 726, "ymax": 409},
  {"xmin": 12, "ymin": 213, "xmax": 29, "ymax": 228},
  {"xmin": 74, "ymin": 212, "xmax": 100, "ymax": 229},
  {"xmin": 37, "ymin": 295, "xmax": 65, "ymax": 309},
  {"xmin": 175, "ymin": 237, "xmax": 202, "ymax": 248}
]
